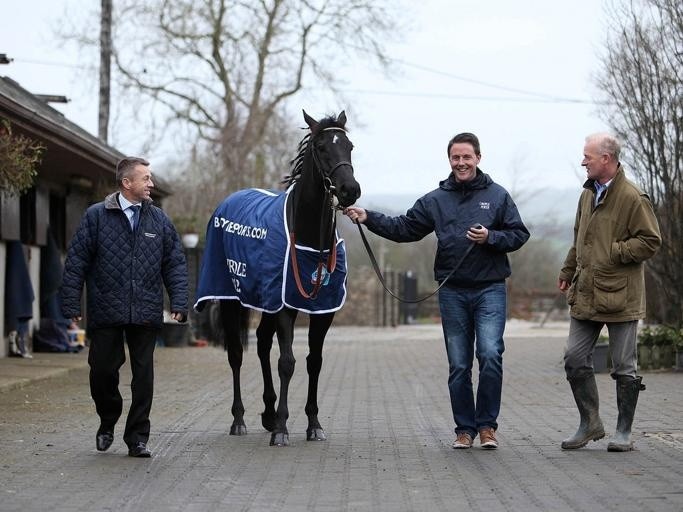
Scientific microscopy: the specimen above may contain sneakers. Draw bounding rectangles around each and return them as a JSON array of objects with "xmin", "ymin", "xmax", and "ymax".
[
  {"xmin": 479, "ymin": 428, "xmax": 498, "ymax": 449},
  {"xmin": 450, "ymin": 432, "xmax": 473, "ymax": 449}
]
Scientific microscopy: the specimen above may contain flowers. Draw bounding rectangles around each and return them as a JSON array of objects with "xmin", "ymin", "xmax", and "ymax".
[{"xmin": 638, "ymin": 327, "xmax": 683, "ymax": 351}]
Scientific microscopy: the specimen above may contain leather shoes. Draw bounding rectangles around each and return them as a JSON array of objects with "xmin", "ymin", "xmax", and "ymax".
[
  {"xmin": 94, "ymin": 422, "xmax": 115, "ymax": 451},
  {"xmin": 125, "ymin": 443, "xmax": 151, "ymax": 457}
]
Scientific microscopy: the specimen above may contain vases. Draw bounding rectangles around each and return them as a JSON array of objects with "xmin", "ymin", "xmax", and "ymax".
[{"xmin": 637, "ymin": 345, "xmax": 683, "ymax": 371}]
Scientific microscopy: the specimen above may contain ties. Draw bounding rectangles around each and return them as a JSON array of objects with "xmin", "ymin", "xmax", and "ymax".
[{"xmin": 128, "ymin": 206, "xmax": 139, "ymax": 237}]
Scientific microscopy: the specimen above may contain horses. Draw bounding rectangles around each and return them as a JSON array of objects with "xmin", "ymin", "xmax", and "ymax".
[{"xmin": 210, "ymin": 110, "xmax": 360, "ymax": 448}]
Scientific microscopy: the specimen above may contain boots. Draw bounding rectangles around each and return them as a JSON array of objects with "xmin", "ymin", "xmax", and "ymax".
[
  {"xmin": 605, "ymin": 371, "xmax": 644, "ymax": 451},
  {"xmin": 560, "ymin": 370, "xmax": 606, "ymax": 449}
]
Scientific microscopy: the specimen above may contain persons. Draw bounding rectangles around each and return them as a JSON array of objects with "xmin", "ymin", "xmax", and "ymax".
[
  {"xmin": 343, "ymin": 132, "xmax": 531, "ymax": 451},
  {"xmin": 556, "ymin": 134, "xmax": 664, "ymax": 454},
  {"xmin": 57, "ymin": 156, "xmax": 190, "ymax": 458}
]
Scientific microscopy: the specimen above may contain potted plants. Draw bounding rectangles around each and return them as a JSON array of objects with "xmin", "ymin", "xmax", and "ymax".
[{"xmin": 591, "ymin": 335, "xmax": 609, "ymax": 372}]
